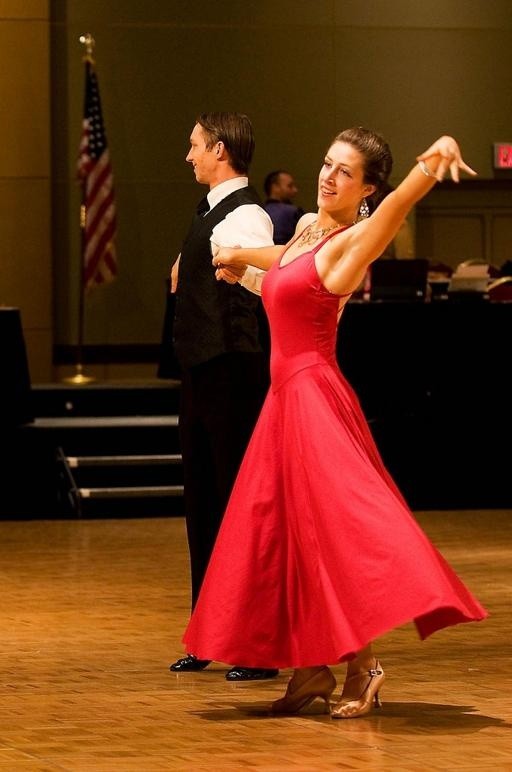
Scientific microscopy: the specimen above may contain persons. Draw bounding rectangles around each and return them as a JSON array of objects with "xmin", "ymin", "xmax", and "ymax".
[
  {"xmin": 257, "ymin": 167, "xmax": 309, "ymax": 249},
  {"xmin": 148, "ymin": 107, "xmax": 280, "ymax": 684},
  {"xmin": 212, "ymin": 122, "xmax": 479, "ymax": 722}
]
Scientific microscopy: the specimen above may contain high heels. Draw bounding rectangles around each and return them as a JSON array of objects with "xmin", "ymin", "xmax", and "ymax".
[
  {"xmin": 271, "ymin": 667, "xmax": 337, "ymax": 714},
  {"xmin": 331, "ymin": 660, "xmax": 386, "ymax": 719}
]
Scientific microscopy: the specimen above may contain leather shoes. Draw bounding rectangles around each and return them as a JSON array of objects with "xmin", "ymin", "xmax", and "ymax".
[
  {"xmin": 224, "ymin": 665, "xmax": 279, "ymax": 682},
  {"xmin": 169, "ymin": 652, "xmax": 212, "ymax": 671}
]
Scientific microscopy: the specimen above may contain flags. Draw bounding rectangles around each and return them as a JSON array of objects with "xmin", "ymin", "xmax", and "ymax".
[{"xmin": 74, "ymin": 54, "xmax": 124, "ymax": 306}]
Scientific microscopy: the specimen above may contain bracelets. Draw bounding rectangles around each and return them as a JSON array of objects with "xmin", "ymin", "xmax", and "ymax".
[{"xmin": 417, "ymin": 158, "xmax": 449, "ymax": 181}]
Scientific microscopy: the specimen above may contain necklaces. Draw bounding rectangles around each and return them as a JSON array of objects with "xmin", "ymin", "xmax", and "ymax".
[{"xmin": 297, "ymin": 219, "xmax": 353, "ymax": 250}]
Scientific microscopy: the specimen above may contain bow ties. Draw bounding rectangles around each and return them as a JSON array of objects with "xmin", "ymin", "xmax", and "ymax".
[{"xmin": 196, "ymin": 196, "xmax": 210, "ymax": 215}]
